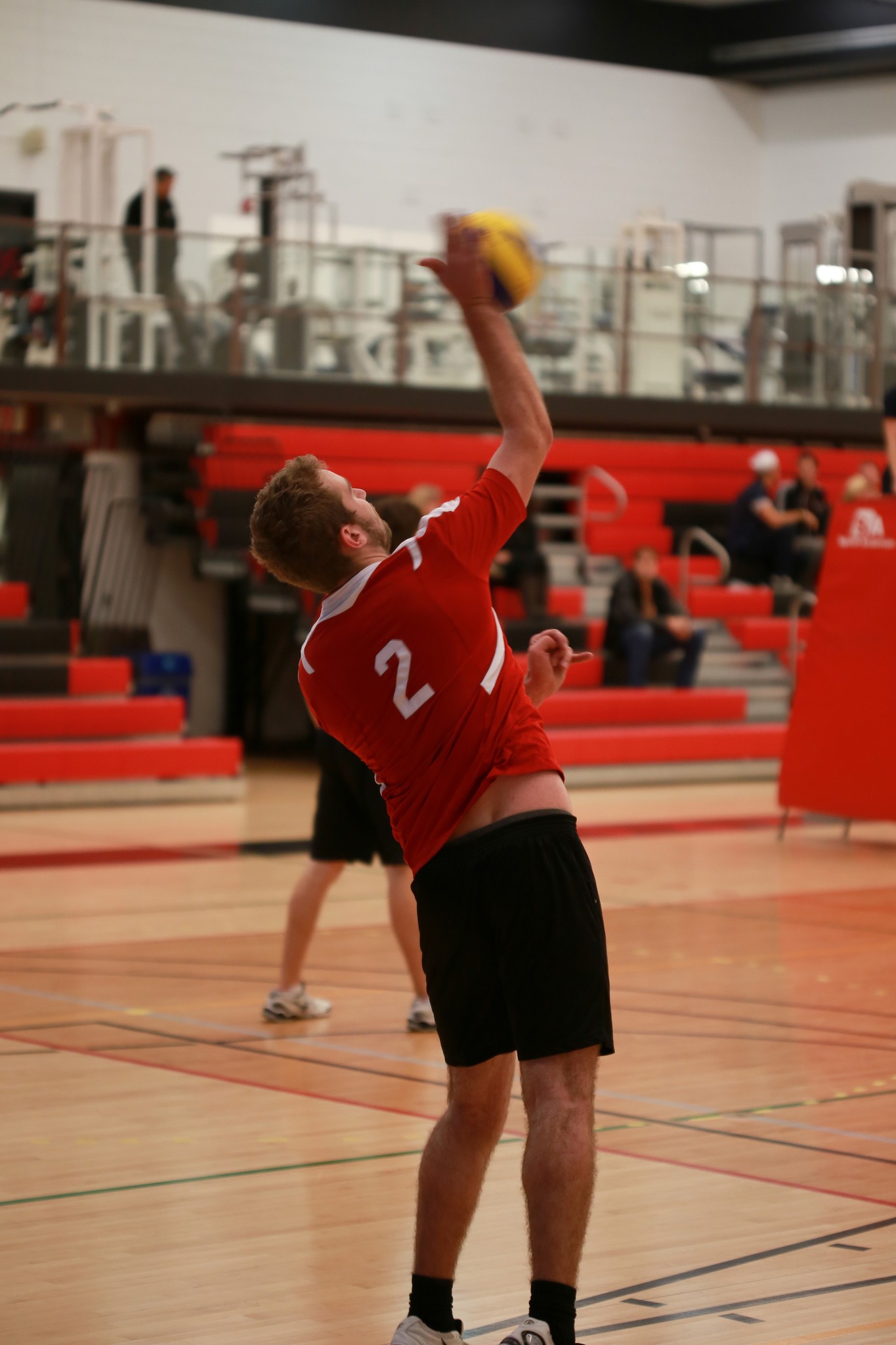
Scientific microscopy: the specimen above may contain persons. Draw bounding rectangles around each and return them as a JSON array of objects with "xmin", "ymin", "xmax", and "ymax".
[
  {"xmin": 122, "ymin": 167, "xmax": 896, "ymax": 1035},
  {"xmin": 253, "ymin": 205, "xmax": 621, "ymax": 1345}
]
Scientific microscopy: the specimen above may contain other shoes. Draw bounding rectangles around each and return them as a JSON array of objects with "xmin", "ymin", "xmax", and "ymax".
[{"xmin": 772, "ymin": 578, "xmax": 817, "ymax": 605}]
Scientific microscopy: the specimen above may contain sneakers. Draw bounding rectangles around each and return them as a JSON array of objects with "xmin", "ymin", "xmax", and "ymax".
[
  {"xmin": 391, "ymin": 1314, "xmax": 469, "ymax": 1345},
  {"xmin": 406, "ymin": 999, "xmax": 440, "ymax": 1032},
  {"xmin": 261, "ymin": 980, "xmax": 333, "ymax": 1021},
  {"xmin": 499, "ymin": 1317, "xmax": 555, "ymax": 1345}
]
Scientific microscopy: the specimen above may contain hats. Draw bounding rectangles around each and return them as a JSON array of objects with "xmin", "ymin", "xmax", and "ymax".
[{"xmin": 751, "ymin": 450, "xmax": 778, "ymax": 474}]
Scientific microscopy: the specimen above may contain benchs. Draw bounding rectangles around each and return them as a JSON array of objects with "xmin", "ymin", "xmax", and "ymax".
[{"xmin": 0, "ymin": 418, "xmax": 895, "ymax": 795}]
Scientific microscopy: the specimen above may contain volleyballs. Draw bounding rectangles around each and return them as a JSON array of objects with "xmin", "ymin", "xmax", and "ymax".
[{"xmin": 459, "ymin": 209, "xmax": 545, "ymax": 312}]
